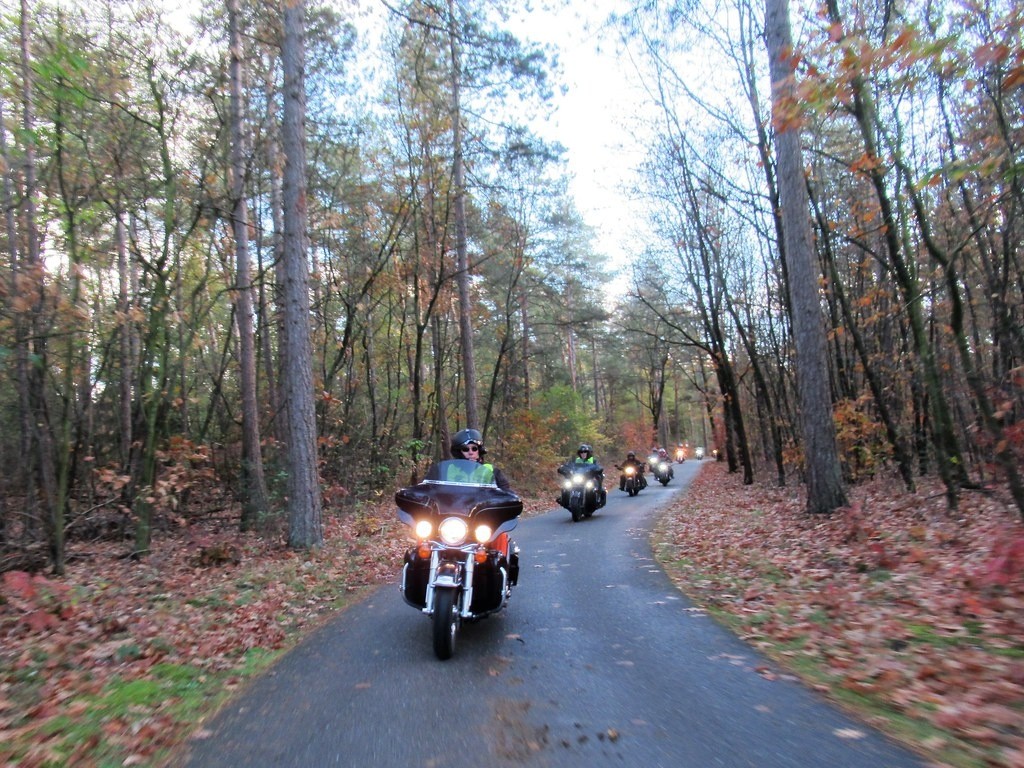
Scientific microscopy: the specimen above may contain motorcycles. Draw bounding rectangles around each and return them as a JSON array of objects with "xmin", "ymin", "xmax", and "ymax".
[
  {"xmin": 394, "ymin": 458, "xmax": 523, "ymax": 659},
  {"xmin": 652, "ymin": 459, "xmax": 674, "ymax": 486},
  {"xmin": 695, "ymin": 449, "xmax": 704, "ymax": 460},
  {"xmin": 647, "ymin": 454, "xmax": 659, "ymax": 472},
  {"xmin": 675, "ymin": 449, "xmax": 685, "ymax": 464},
  {"xmin": 555, "ymin": 463, "xmax": 607, "ymax": 522},
  {"xmin": 613, "ymin": 463, "xmax": 650, "ymax": 496}
]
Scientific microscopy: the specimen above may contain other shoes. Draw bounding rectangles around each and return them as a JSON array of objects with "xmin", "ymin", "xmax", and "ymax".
[{"xmin": 619, "ymin": 487, "xmax": 624, "ymax": 491}]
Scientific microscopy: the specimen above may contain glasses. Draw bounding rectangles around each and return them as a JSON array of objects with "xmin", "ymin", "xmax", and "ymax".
[
  {"xmin": 462, "ymin": 446, "xmax": 479, "ymax": 452},
  {"xmin": 580, "ymin": 451, "xmax": 587, "ymax": 453}
]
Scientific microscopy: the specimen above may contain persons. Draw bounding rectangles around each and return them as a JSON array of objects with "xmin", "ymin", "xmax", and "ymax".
[
  {"xmin": 416, "ymin": 429, "xmax": 511, "ymax": 598},
  {"xmin": 557, "ymin": 443, "xmax": 702, "ymax": 507}
]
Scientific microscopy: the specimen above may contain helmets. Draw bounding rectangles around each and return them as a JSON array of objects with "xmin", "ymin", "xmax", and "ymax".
[
  {"xmin": 578, "ymin": 444, "xmax": 588, "ymax": 451},
  {"xmin": 658, "ymin": 448, "xmax": 665, "ymax": 455},
  {"xmin": 588, "ymin": 445, "xmax": 593, "ymax": 451},
  {"xmin": 451, "ymin": 429, "xmax": 483, "ymax": 447},
  {"xmin": 627, "ymin": 451, "xmax": 635, "ymax": 455},
  {"xmin": 652, "ymin": 448, "xmax": 657, "ymax": 451}
]
